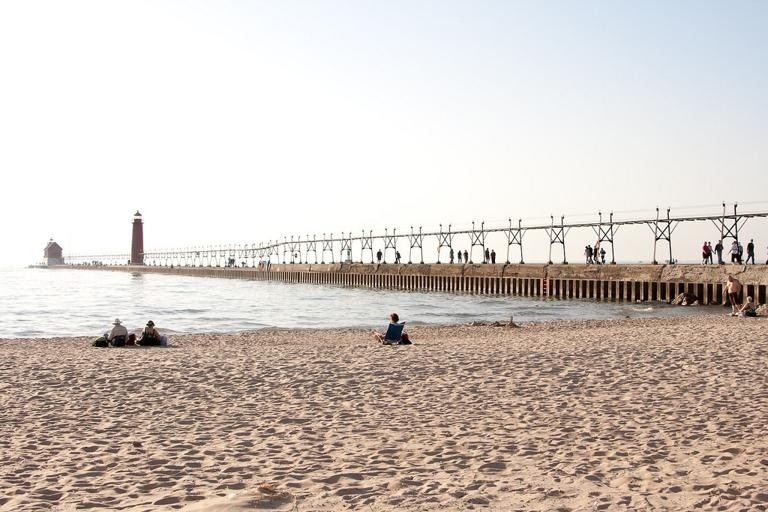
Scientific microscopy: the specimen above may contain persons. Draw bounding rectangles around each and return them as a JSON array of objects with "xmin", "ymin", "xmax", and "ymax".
[
  {"xmin": 723, "ymin": 276, "xmax": 742, "ymax": 315},
  {"xmin": 701, "ymin": 237, "xmax": 756, "ymax": 265},
  {"xmin": 457, "ymin": 250, "xmax": 462, "ymax": 263},
  {"xmin": 485, "ymin": 248, "xmax": 490, "ymax": 263},
  {"xmin": 107, "ymin": 317, "xmax": 128, "ymax": 348},
  {"xmin": 138, "ymin": 320, "xmax": 160, "ymax": 346},
  {"xmin": 490, "ymin": 249, "xmax": 496, "ymax": 264},
  {"xmin": 395, "ymin": 250, "xmax": 401, "ymax": 264},
  {"xmin": 449, "ymin": 249, "xmax": 454, "ymax": 263},
  {"xmin": 739, "ymin": 296, "xmax": 756, "ymax": 317},
  {"xmin": 376, "ymin": 249, "xmax": 382, "ymax": 262},
  {"xmin": 463, "ymin": 250, "xmax": 468, "ymax": 263},
  {"xmin": 583, "ymin": 245, "xmax": 607, "ymax": 264},
  {"xmin": 375, "ymin": 312, "xmax": 400, "ymax": 344}
]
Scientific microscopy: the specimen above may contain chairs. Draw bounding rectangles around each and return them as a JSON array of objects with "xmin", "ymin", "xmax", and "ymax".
[{"xmin": 376, "ymin": 322, "xmax": 406, "ymax": 345}]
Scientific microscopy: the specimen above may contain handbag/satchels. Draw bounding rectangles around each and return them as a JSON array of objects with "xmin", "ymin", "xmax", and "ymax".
[
  {"xmin": 96, "ymin": 337, "xmax": 108, "ymax": 347},
  {"xmin": 127, "ymin": 334, "xmax": 135, "ymax": 345}
]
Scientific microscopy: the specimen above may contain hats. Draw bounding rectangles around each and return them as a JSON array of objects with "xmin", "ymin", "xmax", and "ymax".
[
  {"xmin": 112, "ymin": 318, "xmax": 123, "ymax": 324},
  {"xmin": 146, "ymin": 320, "xmax": 155, "ymax": 326}
]
